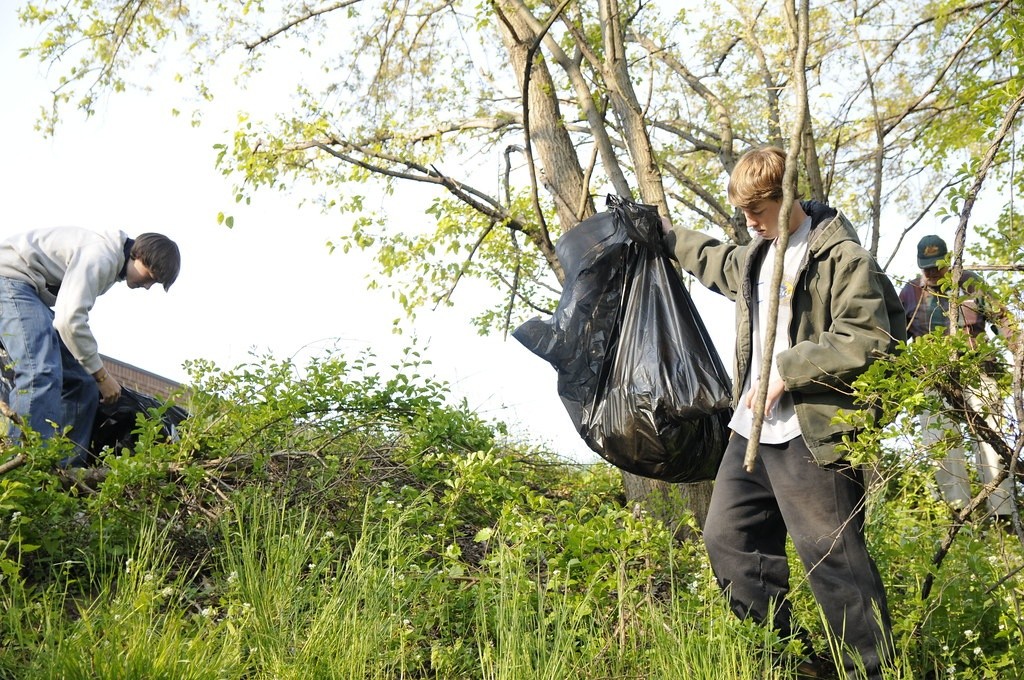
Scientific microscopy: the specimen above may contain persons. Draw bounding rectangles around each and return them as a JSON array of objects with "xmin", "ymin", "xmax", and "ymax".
[
  {"xmin": 899, "ymin": 235, "xmax": 1024, "ymax": 534},
  {"xmin": 663, "ymin": 145, "xmax": 906, "ymax": 680},
  {"xmin": 1, "ymin": 226, "xmax": 180, "ymax": 475}
]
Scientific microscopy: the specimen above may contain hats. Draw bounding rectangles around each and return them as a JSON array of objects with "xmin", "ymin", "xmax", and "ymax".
[{"xmin": 918, "ymin": 234, "xmax": 948, "ymax": 269}]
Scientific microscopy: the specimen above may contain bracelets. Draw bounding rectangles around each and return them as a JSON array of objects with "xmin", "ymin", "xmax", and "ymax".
[{"xmin": 96, "ymin": 371, "xmax": 108, "ymax": 382}]
